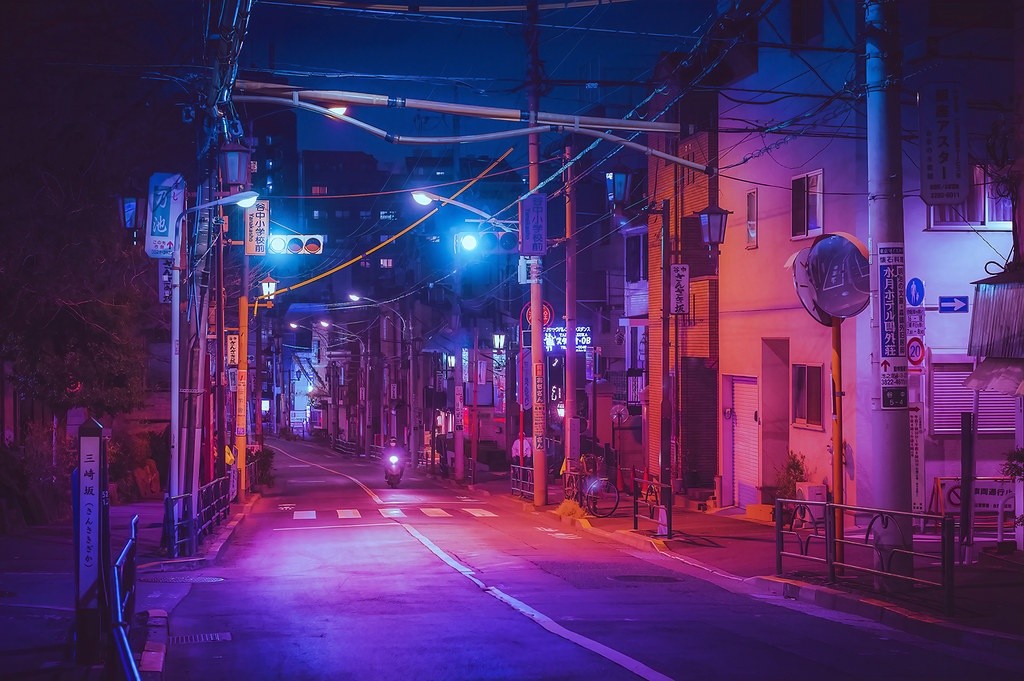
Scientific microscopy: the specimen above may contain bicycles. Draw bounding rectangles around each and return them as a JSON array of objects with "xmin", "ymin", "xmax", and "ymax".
[{"xmin": 566, "ymin": 454, "xmax": 620, "ymax": 518}]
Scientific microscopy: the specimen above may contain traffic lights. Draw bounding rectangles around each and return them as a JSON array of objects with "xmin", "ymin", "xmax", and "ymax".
[
  {"xmin": 455, "ymin": 232, "xmax": 520, "ymax": 256},
  {"xmin": 267, "ymin": 234, "xmax": 323, "ymax": 255}
]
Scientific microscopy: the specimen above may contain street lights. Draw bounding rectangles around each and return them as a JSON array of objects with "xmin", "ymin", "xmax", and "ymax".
[
  {"xmin": 290, "ymin": 323, "xmax": 338, "ymax": 449},
  {"xmin": 167, "ymin": 190, "xmax": 259, "ymax": 560},
  {"xmin": 409, "ymin": 189, "xmax": 547, "ymax": 510},
  {"xmin": 604, "ymin": 167, "xmax": 736, "ymax": 533},
  {"xmin": 321, "ymin": 321, "xmax": 361, "ymax": 456},
  {"xmin": 348, "ymin": 294, "xmax": 417, "ymax": 469}
]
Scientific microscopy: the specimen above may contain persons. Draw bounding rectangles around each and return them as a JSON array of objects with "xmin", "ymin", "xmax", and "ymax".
[
  {"xmin": 512, "ymin": 432, "xmax": 531, "ymax": 483},
  {"xmin": 384, "ymin": 436, "xmax": 406, "ymax": 481},
  {"xmin": 213, "ymin": 431, "xmax": 218, "ymax": 464}
]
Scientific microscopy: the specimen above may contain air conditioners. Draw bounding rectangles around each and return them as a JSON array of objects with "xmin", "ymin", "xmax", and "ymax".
[{"xmin": 795, "ymin": 481, "xmax": 826, "ymax": 520}]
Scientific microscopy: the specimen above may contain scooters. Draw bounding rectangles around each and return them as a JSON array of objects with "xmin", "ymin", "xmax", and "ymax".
[{"xmin": 383, "ymin": 456, "xmax": 403, "ymax": 489}]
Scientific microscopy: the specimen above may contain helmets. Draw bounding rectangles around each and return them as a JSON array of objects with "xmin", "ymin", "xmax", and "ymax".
[{"xmin": 389, "ymin": 437, "xmax": 397, "ymax": 446}]
